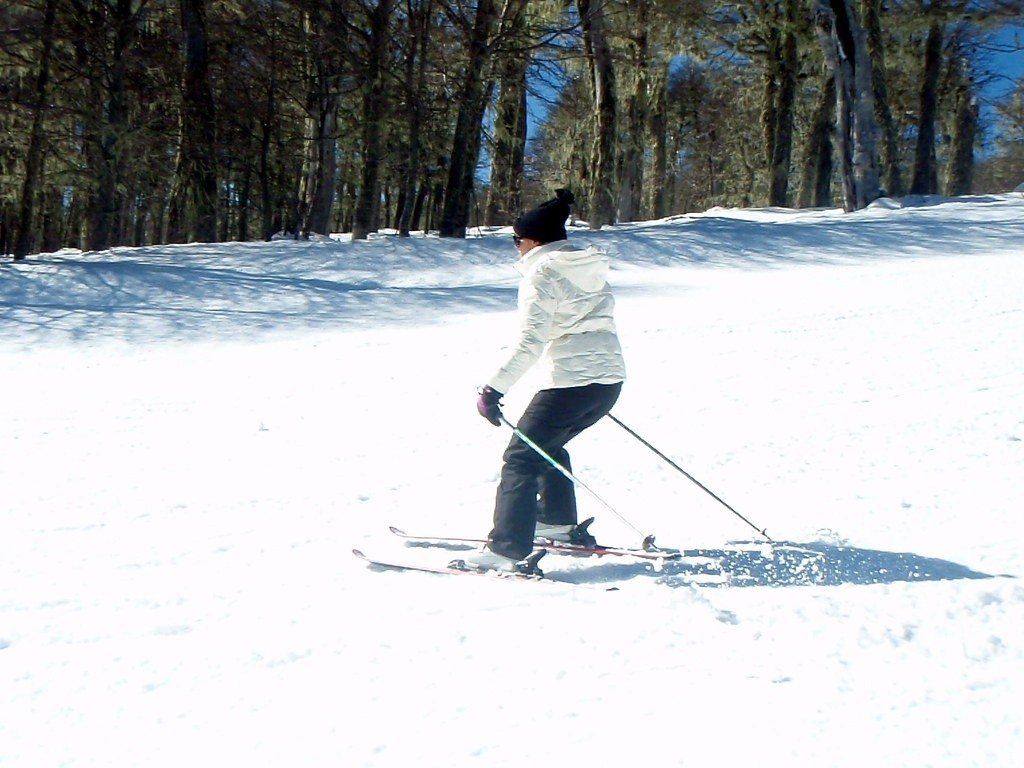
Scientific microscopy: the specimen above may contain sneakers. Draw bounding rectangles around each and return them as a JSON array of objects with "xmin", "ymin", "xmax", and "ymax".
[
  {"xmin": 534, "ymin": 519, "xmax": 577, "ymax": 542},
  {"xmin": 465, "ymin": 544, "xmax": 521, "ymax": 573}
]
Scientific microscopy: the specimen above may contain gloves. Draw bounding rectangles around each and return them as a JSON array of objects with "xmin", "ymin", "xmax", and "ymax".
[{"xmin": 477, "ymin": 384, "xmax": 503, "ymax": 428}]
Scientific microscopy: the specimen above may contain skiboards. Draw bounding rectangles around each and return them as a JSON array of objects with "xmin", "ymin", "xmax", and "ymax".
[
  {"xmin": 352, "ymin": 547, "xmax": 621, "ymax": 592},
  {"xmin": 384, "ymin": 522, "xmax": 682, "ymax": 561}
]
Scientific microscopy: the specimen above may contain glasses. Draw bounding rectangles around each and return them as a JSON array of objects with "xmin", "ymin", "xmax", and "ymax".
[{"xmin": 511, "ymin": 232, "xmax": 524, "ymax": 245}]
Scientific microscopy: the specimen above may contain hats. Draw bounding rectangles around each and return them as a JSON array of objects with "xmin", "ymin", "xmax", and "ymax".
[{"xmin": 513, "ymin": 188, "xmax": 576, "ymax": 243}]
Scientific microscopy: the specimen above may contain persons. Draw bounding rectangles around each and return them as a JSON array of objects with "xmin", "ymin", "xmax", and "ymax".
[{"xmin": 456, "ymin": 187, "xmax": 624, "ymax": 572}]
